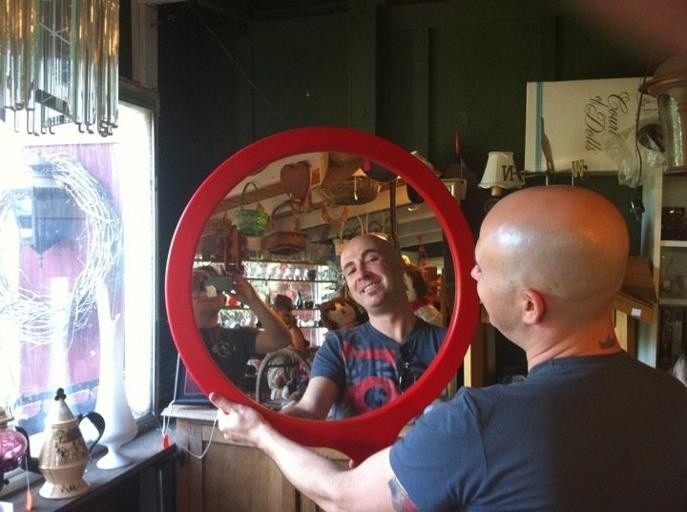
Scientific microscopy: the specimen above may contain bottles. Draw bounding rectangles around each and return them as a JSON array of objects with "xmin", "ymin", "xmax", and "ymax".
[{"xmin": 295, "ymin": 291, "xmax": 303, "ymax": 310}]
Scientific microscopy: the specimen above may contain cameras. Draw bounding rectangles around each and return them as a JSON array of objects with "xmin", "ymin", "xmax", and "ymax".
[{"xmin": 199, "ymin": 275, "xmax": 234, "ymax": 292}]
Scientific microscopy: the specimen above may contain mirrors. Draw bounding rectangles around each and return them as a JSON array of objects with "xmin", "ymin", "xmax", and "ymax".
[{"xmin": 164, "ymin": 130, "xmax": 479, "ymax": 443}]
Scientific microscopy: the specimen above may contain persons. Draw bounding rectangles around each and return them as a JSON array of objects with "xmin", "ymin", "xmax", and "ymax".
[
  {"xmin": 188, "ymin": 264, "xmax": 293, "ymax": 393},
  {"xmin": 208, "ymin": 182, "xmax": 685, "ymax": 510},
  {"xmin": 257, "ymin": 291, "xmax": 312, "ymax": 348},
  {"xmin": 277, "ymin": 234, "xmax": 449, "ymax": 423}
]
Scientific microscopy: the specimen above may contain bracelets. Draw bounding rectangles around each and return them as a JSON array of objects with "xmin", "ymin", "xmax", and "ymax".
[{"xmin": 287, "ymin": 324, "xmax": 294, "ymax": 330}]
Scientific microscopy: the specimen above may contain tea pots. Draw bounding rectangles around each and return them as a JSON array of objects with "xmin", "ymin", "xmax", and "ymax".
[{"xmin": 9, "ymin": 388, "xmax": 106, "ymax": 503}]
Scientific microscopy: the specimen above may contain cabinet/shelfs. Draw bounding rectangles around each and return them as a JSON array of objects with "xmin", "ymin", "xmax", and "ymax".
[
  {"xmin": 642, "ymin": 167, "xmax": 686, "ymax": 377},
  {"xmin": 163, "ymin": 416, "xmax": 352, "ymax": 512},
  {"xmin": 190, "ymin": 254, "xmax": 344, "ymax": 350}
]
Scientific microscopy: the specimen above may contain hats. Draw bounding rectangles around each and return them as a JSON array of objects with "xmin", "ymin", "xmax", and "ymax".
[{"xmin": 269, "ymin": 295, "xmax": 297, "ymax": 309}]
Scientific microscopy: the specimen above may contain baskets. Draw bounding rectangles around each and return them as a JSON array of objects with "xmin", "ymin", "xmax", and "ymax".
[
  {"xmin": 278, "ymin": 244, "xmax": 332, "ymax": 266},
  {"xmin": 234, "ymin": 183, "xmax": 268, "ymax": 236},
  {"xmin": 313, "ymin": 176, "xmax": 381, "ymax": 206},
  {"xmin": 202, "ymin": 210, "xmax": 232, "ymax": 242},
  {"xmin": 263, "ymin": 204, "xmax": 308, "ymax": 256}
]
